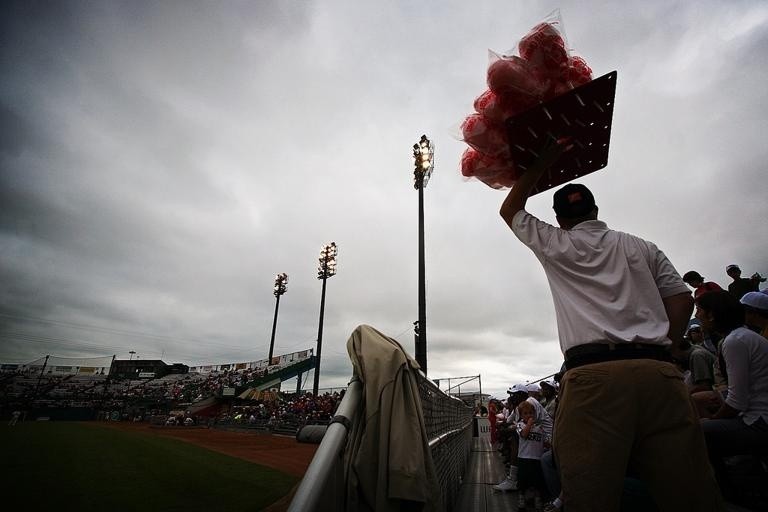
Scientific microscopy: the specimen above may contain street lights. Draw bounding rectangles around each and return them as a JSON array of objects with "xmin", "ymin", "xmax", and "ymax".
[
  {"xmin": 413, "ymin": 134, "xmax": 435, "ymax": 375},
  {"xmin": 269, "ymin": 273, "xmax": 287, "ymax": 357},
  {"xmin": 312, "ymin": 242, "xmax": 338, "ymax": 395}
]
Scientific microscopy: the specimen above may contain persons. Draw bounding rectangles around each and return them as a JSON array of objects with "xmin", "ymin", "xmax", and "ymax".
[
  {"xmin": 486, "ymin": 362, "xmax": 568, "ymax": 512},
  {"xmin": 1, "ymin": 354, "xmax": 342, "ymax": 432},
  {"xmin": 499, "ymin": 140, "xmax": 719, "ymax": 509},
  {"xmin": 667, "ymin": 264, "xmax": 768, "ymax": 512}
]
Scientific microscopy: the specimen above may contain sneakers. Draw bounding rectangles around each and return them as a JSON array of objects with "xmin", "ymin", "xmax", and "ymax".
[
  {"xmin": 544, "ymin": 500, "xmax": 561, "ymax": 512},
  {"xmin": 493, "ymin": 476, "xmax": 521, "ymax": 492}
]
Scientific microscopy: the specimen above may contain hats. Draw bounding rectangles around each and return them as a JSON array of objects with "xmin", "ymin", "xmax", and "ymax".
[
  {"xmin": 739, "ymin": 292, "xmax": 768, "ymax": 310},
  {"xmin": 507, "ymin": 381, "xmax": 558, "ymax": 393}
]
